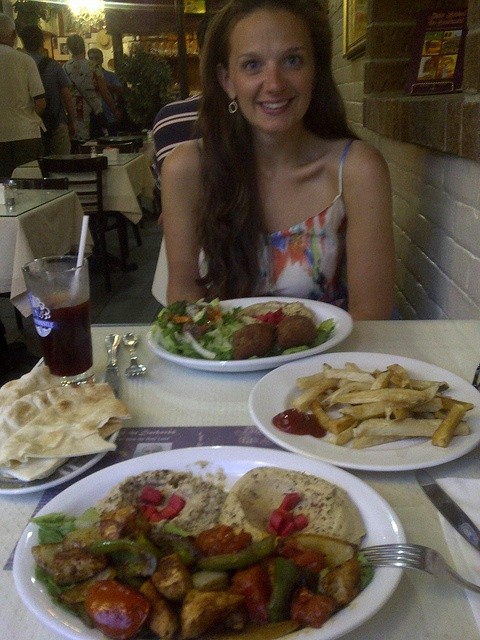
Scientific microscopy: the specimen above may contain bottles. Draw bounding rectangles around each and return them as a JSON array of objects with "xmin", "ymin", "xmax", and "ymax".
[{"xmin": 90, "ymin": 146, "xmax": 97, "ymax": 159}]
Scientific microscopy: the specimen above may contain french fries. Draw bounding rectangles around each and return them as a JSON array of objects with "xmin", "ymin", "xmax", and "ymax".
[{"xmin": 293, "ymin": 361, "xmax": 474, "ymax": 448}]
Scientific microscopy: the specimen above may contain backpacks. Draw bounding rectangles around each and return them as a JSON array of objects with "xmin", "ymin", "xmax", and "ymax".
[{"xmin": 37, "ymin": 57, "xmax": 62, "ymax": 131}]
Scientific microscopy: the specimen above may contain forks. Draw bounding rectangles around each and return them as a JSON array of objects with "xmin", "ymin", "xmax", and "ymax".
[
  {"xmin": 122, "ymin": 333, "xmax": 147, "ymax": 377},
  {"xmin": 357, "ymin": 543, "xmax": 480, "ymax": 594}
]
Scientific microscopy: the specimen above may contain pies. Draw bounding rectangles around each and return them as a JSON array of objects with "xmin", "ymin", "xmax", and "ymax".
[{"xmin": 0, "ymin": 359, "xmax": 133, "ymax": 466}]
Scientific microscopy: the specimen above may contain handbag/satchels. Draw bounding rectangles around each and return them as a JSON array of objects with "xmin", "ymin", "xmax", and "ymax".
[{"xmin": 89, "ymin": 112, "xmax": 107, "ymax": 127}]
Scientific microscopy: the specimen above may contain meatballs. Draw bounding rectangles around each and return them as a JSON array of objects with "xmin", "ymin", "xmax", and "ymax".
[
  {"xmin": 275, "ymin": 315, "xmax": 316, "ymax": 349},
  {"xmin": 233, "ymin": 322, "xmax": 275, "ymax": 360}
]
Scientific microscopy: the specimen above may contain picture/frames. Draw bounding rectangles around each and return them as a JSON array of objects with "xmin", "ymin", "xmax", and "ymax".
[
  {"xmin": 51, "ymin": 36, "xmax": 70, "ymax": 61},
  {"xmin": 343, "ymin": 0, "xmax": 373, "ymax": 58}
]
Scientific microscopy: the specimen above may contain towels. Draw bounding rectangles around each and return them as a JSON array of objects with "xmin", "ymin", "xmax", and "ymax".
[{"xmin": 435, "ymin": 475, "xmax": 479, "ymax": 628}]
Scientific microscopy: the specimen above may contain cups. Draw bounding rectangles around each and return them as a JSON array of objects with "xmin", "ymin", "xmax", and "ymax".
[
  {"xmin": 20, "ymin": 256, "xmax": 96, "ymax": 388},
  {"xmin": 3, "ymin": 180, "xmax": 17, "ymax": 206}
]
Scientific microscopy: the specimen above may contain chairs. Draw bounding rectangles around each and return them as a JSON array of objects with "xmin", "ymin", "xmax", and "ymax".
[
  {"xmin": 15, "ymin": 177, "xmax": 68, "ymax": 190},
  {"xmin": 78, "ymin": 142, "xmax": 134, "ymax": 153},
  {"xmin": 38, "ymin": 155, "xmax": 128, "ymax": 270}
]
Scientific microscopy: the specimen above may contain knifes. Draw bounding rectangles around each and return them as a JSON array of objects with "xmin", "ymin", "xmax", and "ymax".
[
  {"xmin": 100, "ymin": 333, "xmax": 120, "ymax": 402},
  {"xmin": 416, "ymin": 471, "xmax": 480, "ymax": 554}
]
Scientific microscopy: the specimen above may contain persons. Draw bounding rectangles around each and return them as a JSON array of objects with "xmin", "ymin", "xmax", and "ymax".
[
  {"xmin": 0, "ymin": 12, "xmax": 47, "ymax": 183},
  {"xmin": 150, "ymin": 8, "xmax": 222, "ymax": 229},
  {"xmin": 17, "ymin": 23, "xmax": 79, "ymax": 173},
  {"xmin": 87, "ymin": 47, "xmax": 125, "ymax": 136},
  {"xmin": 62, "ymin": 35, "xmax": 126, "ymax": 153},
  {"xmin": 151, "ymin": 0, "xmax": 397, "ymax": 322}
]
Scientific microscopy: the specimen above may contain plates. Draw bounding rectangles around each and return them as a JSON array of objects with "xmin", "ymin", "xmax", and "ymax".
[
  {"xmin": 147, "ymin": 296, "xmax": 354, "ymax": 372},
  {"xmin": 12, "ymin": 445, "xmax": 407, "ymax": 640},
  {"xmin": 248, "ymin": 352, "xmax": 480, "ymax": 472},
  {"xmin": 0, "ymin": 422, "xmax": 120, "ymax": 494}
]
